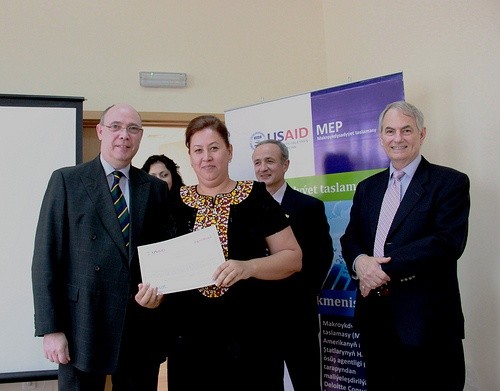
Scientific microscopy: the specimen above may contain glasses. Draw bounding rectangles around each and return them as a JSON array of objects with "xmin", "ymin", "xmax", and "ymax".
[{"xmin": 103, "ymin": 124, "xmax": 142, "ymax": 134}]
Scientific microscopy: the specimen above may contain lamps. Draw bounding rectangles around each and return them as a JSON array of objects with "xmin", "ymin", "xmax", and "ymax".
[{"xmin": 140, "ymin": 72, "xmax": 186, "ymax": 87}]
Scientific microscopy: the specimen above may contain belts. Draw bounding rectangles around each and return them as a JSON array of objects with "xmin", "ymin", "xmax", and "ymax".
[{"xmin": 369, "ymin": 286, "xmax": 394, "ymax": 299}]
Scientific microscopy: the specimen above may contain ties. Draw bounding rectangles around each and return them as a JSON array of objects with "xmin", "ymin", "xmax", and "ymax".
[
  {"xmin": 110, "ymin": 171, "xmax": 131, "ymax": 250},
  {"xmin": 373, "ymin": 170, "xmax": 404, "ymax": 270}
]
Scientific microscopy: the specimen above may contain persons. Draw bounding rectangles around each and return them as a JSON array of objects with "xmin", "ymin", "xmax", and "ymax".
[
  {"xmin": 134, "ymin": 115, "xmax": 303, "ymax": 391},
  {"xmin": 31, "ymin": 104, "xmax": 175, "ymax": 391},
  {"xmin": 140, "ymin": 153, "xmax": 191, "ymax": 391},
  {"xmin": 252, "ymin": 139, "xmax": 334, "ymax": 391},
  {"xmin": 340, "ymin": 102, "xmax": 471, "ymax": 391}
]
